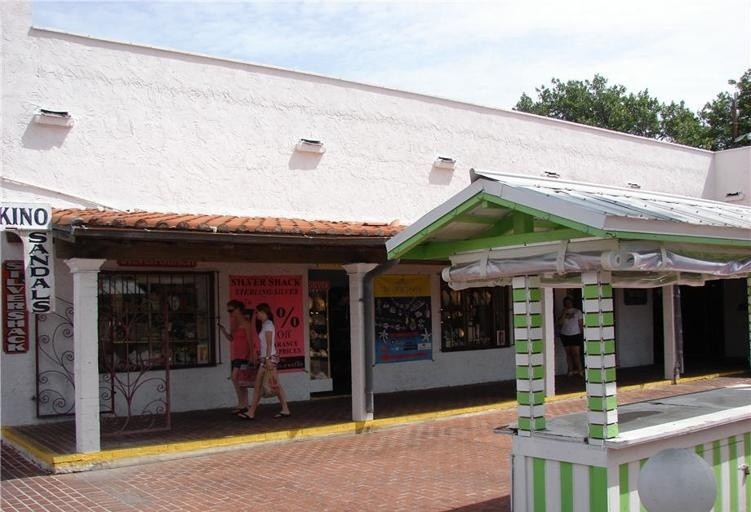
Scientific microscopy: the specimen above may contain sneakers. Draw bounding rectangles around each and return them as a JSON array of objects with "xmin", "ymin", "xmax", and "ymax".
[{"xmin": 231, "ymin": 406, "xmax": 251, "ymax": 414}]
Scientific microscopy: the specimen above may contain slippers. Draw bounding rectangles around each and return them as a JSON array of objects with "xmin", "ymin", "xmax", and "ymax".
[
  {"xmin": 237, "ymin": 412, "xmax": 256, "ymax": 420},
  {"xmin": 273, "ymin": 412, "xmax": 292, "ymax": 419}
]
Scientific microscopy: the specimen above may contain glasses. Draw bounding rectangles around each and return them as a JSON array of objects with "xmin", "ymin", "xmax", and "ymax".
[{"xmin": 227, "ymin": 306, "xmax": 237, "ymax": 314}]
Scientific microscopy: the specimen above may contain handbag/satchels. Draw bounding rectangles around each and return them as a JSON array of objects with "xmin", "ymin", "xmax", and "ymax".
[
  {"xmin": 260, "ymin": 358, "xmax": 282, "ymax": 398},
  {"xmin": 238, "ymin": 359, "xmax": 258, "ymax": 387}
]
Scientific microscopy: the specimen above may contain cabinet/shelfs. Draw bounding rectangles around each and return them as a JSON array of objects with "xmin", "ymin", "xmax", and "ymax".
[
  {"xmin": 308, "ymin": 280, "xmax": 333, "ymax": 393},
  {"xmin": 112, "ymin": 311, "xmax": 197, "ymax": 344}
]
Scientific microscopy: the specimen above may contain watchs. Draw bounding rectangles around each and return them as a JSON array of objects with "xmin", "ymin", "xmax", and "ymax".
[{"xmin": 265, "ymin": 356, "xmax": 272, "ymax": 360}]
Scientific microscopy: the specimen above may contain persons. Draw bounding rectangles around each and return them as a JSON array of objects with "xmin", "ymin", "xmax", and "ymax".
[
  {"xmin": 235, "ymin": 303, "xmax": 291, "ymax": 420},
  {"xmin": 216, "ymin": 300, "xmax": 254, "ymax": 414},
  {"xmin": 556, "ymin": 295, "xmax": 585, "ymax": 379}
]
